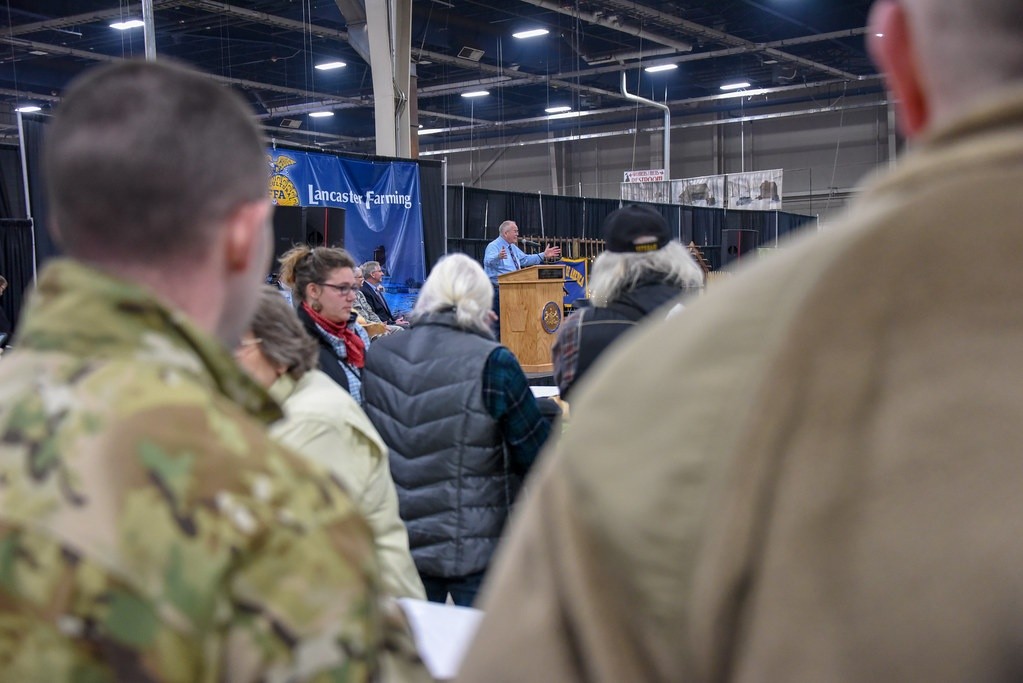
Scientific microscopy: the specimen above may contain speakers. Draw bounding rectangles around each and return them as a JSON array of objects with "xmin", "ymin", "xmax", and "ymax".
[
  {"xmin": 268, "ymin": 206, "xmax": 345, "ymax": 279},
  {"xmin": 721, "ymin": 229, "xmax": 759, "ymax": 268}
]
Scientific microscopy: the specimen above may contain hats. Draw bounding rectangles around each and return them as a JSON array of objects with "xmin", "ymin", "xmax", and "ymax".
[{"xmin": 604, "ymin": 202, "xmax": 671, "ymax": 253}]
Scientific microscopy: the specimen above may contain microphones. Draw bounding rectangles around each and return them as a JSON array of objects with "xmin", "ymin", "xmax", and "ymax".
[{"xmin": 522, "ymin": 239, "xmax": 542, "ymax": 247}]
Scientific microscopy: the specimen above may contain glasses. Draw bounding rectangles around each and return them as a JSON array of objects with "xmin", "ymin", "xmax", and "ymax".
[{"xmin": 303, "ymin": 281, "xmax": 359, "ymax": 296}]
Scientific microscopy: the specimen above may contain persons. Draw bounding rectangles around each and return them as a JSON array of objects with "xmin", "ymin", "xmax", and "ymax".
[
  {"xmin": 456, "ymin": 0, "xmax": 908, "ymax": 683},
  {"xmin": 230, "ymin": 203, "xmax": 705, "ymax": 610},
  {"xmin": 1, "ymin": 62, "xmax": 430, "ymax": 682}
]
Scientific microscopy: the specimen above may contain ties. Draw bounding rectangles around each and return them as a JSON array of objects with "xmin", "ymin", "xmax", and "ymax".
[
  {"xmin": 376, "ymin": 288, "xmax": 389, "ymax": 312},
  {"xmin": 508, "ymin": 246, "xmax": 520, "ymax": 270}
]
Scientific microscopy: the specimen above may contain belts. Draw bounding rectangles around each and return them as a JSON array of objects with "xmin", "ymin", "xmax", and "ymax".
[{"xmin": 493, "ymin": 285, "xmax": 499, "ymax": 288}]
